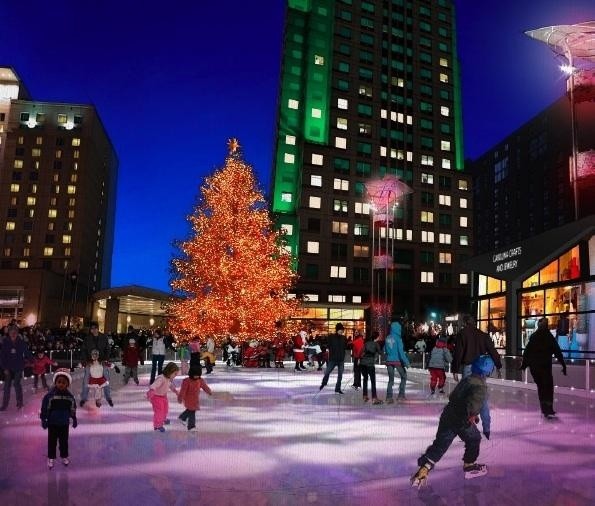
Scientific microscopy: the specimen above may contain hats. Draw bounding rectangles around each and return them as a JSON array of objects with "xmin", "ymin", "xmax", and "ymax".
[
  {"xmin": 335, "ymin": 323, "xmax": 345, "ymax": 331},
  {"xmin": 53, "ymin": 367, "xmax": 72, "ymax": 385},
  {"xmin": 470, "ymin": 355, "xmax": 494, "ymax": 377}
]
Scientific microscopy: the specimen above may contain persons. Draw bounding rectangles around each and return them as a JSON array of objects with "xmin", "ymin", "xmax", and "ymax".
[
  {"xmin": 1, "ymin": 325, "xmax": 33, "ymax": 411},
  {"xmin": 293, "ymin": 322, "xmax": 410, "ymax": 404},
  {"xmin": 177, "ymin": 363, "xmax": 213, "ymax": 432},
  {"xmin": 414, "ymin": 334, "xmax": 453, "ymax": 394},
  {"xmin": 451, "ymin": 316, "xmax": 503, "ymax": 440},
  {"xmin": 1, "ymin": 316, "xmax": 177, "ymax": 386},
  {"xmin": 79, "ymin": 320, "xmax": 120, "ymax": 407},
  {"xmin": 179, "ymin": 334, "xmax": 285, "ymax": 375},
  {"xmin": 409, "ymin": 356, "xmax": 495, "ymax": 488},
  {"xmin": 40, "ymin": 368, "xmax": 78, "ymax": 470},
  {"xmin": 518, "ymin": 318, "xmax": 566, "ymax": 416},
  {"xmin": 146, "ymin": 362, "xmax": 180, "ymax": 433}
]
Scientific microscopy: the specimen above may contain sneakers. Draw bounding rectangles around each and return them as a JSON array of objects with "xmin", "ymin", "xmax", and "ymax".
[
  {"xmin": 47, "ymin": 457, "xmax": 55, "ymax": 468},
  {"xmin": 176, "ymin": 417, "xmax": 187, "ymax": 425},
  {"xmin": 462, "ymin": 461, "xmax": 486, "ymax": 472},
  {"xmin": 62, "ymin": 457, "xmax": 69, "ymax": 465},
  {"xmin": 155, "ymin": 427, "xmax": 165, "ymax": 433},
  {"xmin": 96, "ymin": 399, "xmax": 101, "ymax": 407},
  {"xmin": 410, "ymin": 463, "xmax": 431, "ymax": 480},
  {"xmin": 363, "ymin": 396, "xmax": 407, "ymax": 405},
  {"xmin": 186, "ymin": 427, "xmax": 199, "ymax": 433}
]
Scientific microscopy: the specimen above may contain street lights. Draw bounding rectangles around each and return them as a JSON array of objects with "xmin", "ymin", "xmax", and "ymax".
[
  {"xmin": 367, "ymin": 175, "xmax": 415, "ymax": 343},
  {"xmin": 523, "ymin": 19, "xmax": 594, "ymax": 222}
]
